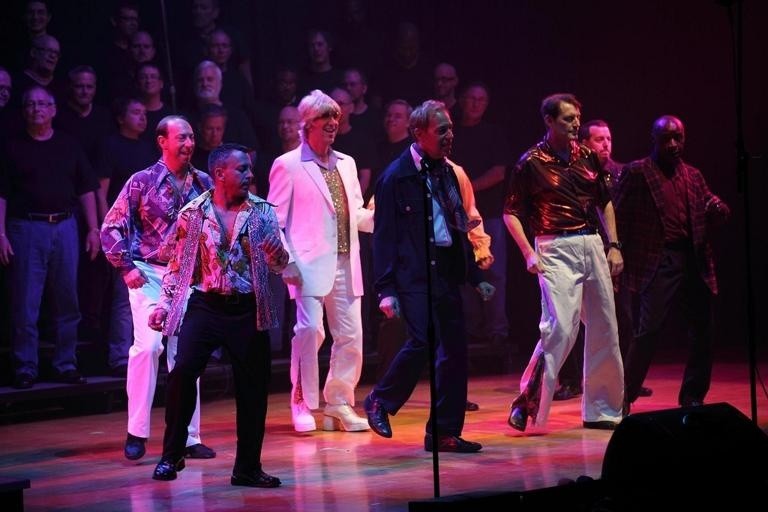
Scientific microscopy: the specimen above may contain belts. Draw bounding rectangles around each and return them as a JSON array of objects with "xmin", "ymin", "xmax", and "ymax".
[
  {"xmin": 23, "ymin": 212, "xmax": 68, "ymax": 223},
  {"xmin": 553, "ymin": 228, "xmax": 598, "ymax": 235},
  {"xmin": 193, "ymin": 289, "xmax": 251, "ymax": 304},
  {"xmin": 132, "ymin": 257, "xmax": 167, "ymax": 267}
]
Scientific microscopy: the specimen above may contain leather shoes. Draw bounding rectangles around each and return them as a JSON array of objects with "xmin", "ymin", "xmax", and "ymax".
[
  {"xmin": 364, "ymin": 391, "xmax": 395, "ymax": 439},
  {"xmin": 639, "ymin": 386, "xmax": 652, "ymax": 396},
  {"xmin": 509, "ymin": 404, "xmax": 529, "ymax": 431},
  {"xmin": 102, "ymin": 363, "xmax": 127, "ymax": 378},
  {"xmin": 11, "ymin": 372, "xmax": 35, "ymax": 388},
  {"xmin": 289, "ymin": 398, "xmax": 317, "ymax": 432},
  {"xmin": 228, "ymin": 465, "xmax": 281, "ymax": 489},
  {"xmin": 322, "ymin": 404, "xmax": 372, "ymax": 433},
  {"xmin": 622, "ymin": 401, "xmax": 631, "ymax": 417},
  {"xmin": 152, "ymin": 454, "xmax": 184, "ymax": 479},
  {"xmin": 53, "ymin": 371, "xmax": 87, "ymax": 384},
  {"xmin": 424, "ymin": 430, "xmax": 483, "ymax": 452},
  {"xmin": 185, "ymin": 443, "xmax": 215, "ymax": 459},
  {"xmin": 466, "ymin": 399, "xmax": 478, "ymax": 413},
  {"xmin": 584, "ymin": 416, "xmax": 618, "ymax": 430},
  {"xmin": 681, "ymin": 399, "xmax": 703, "ymax": 407},
  {"xmin": 124, "ymin": 432, "xmax": 147, "ymax": 459},
  {"xmin": 551, "ymin": 384, "xmax": 584, "ymax": 400}
]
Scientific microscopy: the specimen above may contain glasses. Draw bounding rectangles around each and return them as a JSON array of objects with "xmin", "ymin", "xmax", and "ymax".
[{"xmin": 313, "ymin": 111, "xmax": 340, "ymax": 121}]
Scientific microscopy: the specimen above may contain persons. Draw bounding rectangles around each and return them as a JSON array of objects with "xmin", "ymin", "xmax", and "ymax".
[
  {"xmin": 610, "ymin": 115, "xmax": 732, "ymax": 418},
  {"xmin": 502, "ymin": 93, "xmax": 625, "ymax": 430},
  {"xmin": 549, "ymin": 121, "xmax": 651, "ymax": 400}
]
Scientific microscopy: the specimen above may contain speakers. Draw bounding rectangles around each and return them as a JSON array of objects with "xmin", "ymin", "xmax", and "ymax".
[{"xmin": 601, "ymin": 402, "xmax": 768, "ymax": 512}]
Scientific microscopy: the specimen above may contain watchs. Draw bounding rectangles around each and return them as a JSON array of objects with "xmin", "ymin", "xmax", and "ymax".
[{"xmin": 607, "ymin": 241, "xmax": 623, "ymax": 249}]
